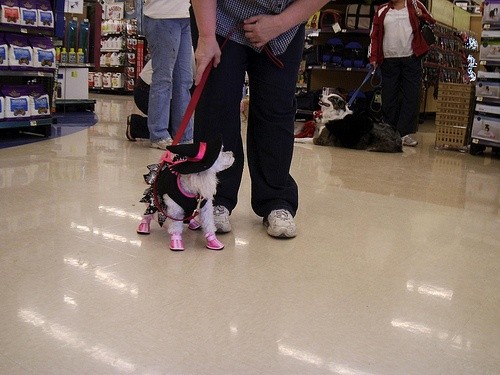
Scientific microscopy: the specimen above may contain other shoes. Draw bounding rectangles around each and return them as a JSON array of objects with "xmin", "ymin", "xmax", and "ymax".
[{"xmin": 126, "ymin": 114, "xmax": 135, "ymax": 141}]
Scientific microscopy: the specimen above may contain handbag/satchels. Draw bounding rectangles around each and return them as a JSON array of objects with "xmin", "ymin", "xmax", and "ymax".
[{"xmin": 419, "ymin": 21, "xmax": 437, "ymax": 45}]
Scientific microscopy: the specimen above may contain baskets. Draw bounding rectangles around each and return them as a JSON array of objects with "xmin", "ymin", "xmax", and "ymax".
[{"xmin": 434, "ymin": 81, "xmax": 475, "ymax": 151}]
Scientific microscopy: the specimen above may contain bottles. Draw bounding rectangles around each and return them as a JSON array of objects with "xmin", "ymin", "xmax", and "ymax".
[
  {"xmin": 55, "ymin": 47, "xmax": 89, "ymax": 64},
  {"xmin": 88, "ymin": 71, "xmax": 126, "ymax": 88},
  {"xmin": 99, "ymin": 19, "xmax": 126, "ymax": 66}
]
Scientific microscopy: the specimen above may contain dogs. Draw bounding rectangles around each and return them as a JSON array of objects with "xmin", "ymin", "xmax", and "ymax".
[
  {"xmin": 312, "ymin": 93, "xmax": 404, "ymax": 153},
  {"xmin": 137, "ymin": 133, "xmax": 236, "ymax": 251}
]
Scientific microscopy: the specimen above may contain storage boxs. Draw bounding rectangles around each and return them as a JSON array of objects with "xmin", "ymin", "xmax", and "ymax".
[{"xmin": 435, "ymin": 82, "xmax": 474, "ymax": 153}]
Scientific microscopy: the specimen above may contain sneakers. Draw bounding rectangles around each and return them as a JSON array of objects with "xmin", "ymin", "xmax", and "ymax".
[
  {"xmin": 402, "ymin": 135, "xmax": 418, "ymax": 147},
  {"xmin": 263, "ymin": 208, "xmax": 297, "ymax": 238},
  {"xmin": 150, "ymin": 137, "xmax": 179, "ymax": 150},
  {"xmin": 213, "ymin": 205, "xmax": 232, "ymax": 232},
  {"xmin": 177, "ymin": 139, "xmax": 193, "ymax": 145}
]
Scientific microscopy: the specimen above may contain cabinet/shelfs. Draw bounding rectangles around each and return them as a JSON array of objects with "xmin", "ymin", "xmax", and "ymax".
[
  {"xmin": 296, "ymin": 0, "xmax": 385, "ymax": 114},
  {"xmin": 473, "ymin": 0, "xmax": 500, "ymax": 149},
  {"xmin": 427, "ymin": 0, "xmax": 483, "ymax": 32},
  {"xmin": 0, "ymin": 0, "xmax": 146, "ymax": 134}
]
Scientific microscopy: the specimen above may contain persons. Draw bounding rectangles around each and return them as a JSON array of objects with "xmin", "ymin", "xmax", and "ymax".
[
  {"xmin": 123, "ymin": 46, "xmax": 195, "ymax": 142},
  {"xmin": 368, "ymin": 0, "xmax": 436, "ymax": 147},
  {"xmin": 187, "ymin": 0, "xmax": 331, "ymax": 239},
  {"xmin": 140, "ymin": 0, "xmax": 196, "ymax": 149}
]
